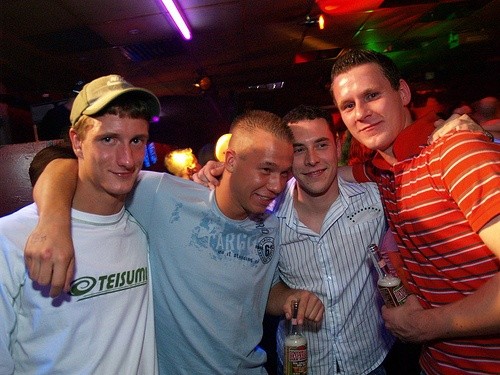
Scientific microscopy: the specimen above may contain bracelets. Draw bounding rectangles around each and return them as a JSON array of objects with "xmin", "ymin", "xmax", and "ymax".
[{"xmin": 485, "ymin": 129, "xmax": 495, "ymax": 143}]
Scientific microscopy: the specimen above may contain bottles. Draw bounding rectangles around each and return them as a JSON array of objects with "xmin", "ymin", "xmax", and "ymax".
[
  {"xmin": 284, "ymin": 300, "xmax": 308, "ymax": 375},
  {"xmin": 367, "ymin": 244, "xmax": 408, "ymax": 340}
]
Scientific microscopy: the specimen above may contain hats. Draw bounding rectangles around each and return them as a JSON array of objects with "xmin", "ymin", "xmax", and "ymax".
[{"xmin": 70, "ymin": 75, "xmax": 161, "ymax": 125}]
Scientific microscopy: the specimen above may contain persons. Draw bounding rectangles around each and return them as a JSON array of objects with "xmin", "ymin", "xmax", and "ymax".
[
  {"xmin": 0, "ymin": 74, "xmax": 163, "ymax": 375},
  {"xmin": 23, "ymin": 110, "xmax": 325, "ymax": 375},
  {"xmin": 267, "ymin": 105, "xmax": 500, "ymax": 375},
  {"xmin": 192, "ymin": 47, "xmax": 500, "ymax": 375},
  {"xmin": 409, "ymin": 88, "xmax": 500, "ymax": 143}
]
